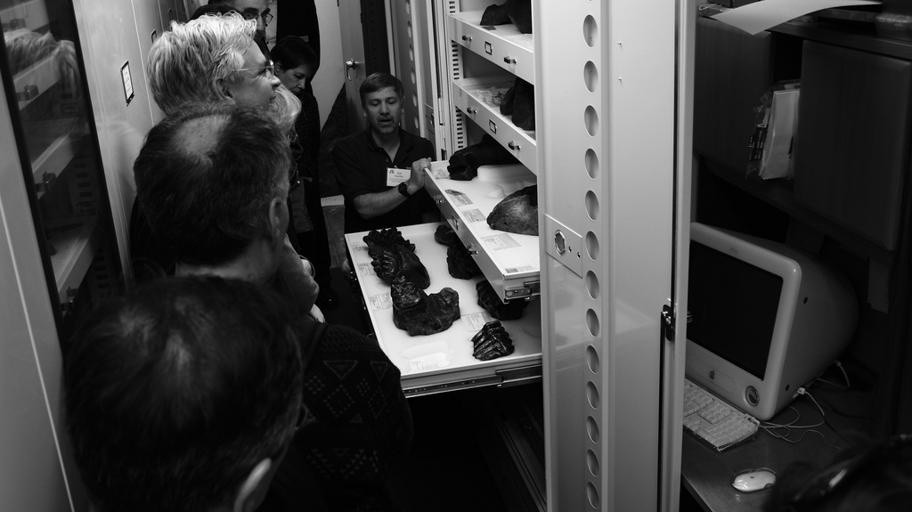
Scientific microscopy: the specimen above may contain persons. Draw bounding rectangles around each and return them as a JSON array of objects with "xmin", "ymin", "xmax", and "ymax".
[{"xmin": 58, "ymin": 0, "xmax": 436, "ymax": 512}]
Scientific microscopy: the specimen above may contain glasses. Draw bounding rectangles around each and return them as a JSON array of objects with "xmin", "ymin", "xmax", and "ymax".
[{"xmin": 233, "ymin": 59, "xmax": 276, "ymax": 78}]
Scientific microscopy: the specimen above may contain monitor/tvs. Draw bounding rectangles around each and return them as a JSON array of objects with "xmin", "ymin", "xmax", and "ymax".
[{"xmin": 685, "ymin": 219, "xmax": 861, "ymax": 421}]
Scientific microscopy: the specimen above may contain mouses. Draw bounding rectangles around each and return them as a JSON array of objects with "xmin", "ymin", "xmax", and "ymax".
[{"xmin": 730, "ymin": 466, "xmax": 776, "ymax": 493}]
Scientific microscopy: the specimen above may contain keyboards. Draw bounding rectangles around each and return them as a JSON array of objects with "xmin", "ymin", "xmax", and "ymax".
[{"xmin": 683, "ymin": 379, "xmax": 760, "ymax": 453}]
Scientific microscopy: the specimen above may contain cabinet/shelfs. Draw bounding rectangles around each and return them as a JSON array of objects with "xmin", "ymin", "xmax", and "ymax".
[{"xmin": 0, "ymin": 0, "xmax": 550, "ymax": 512}]
